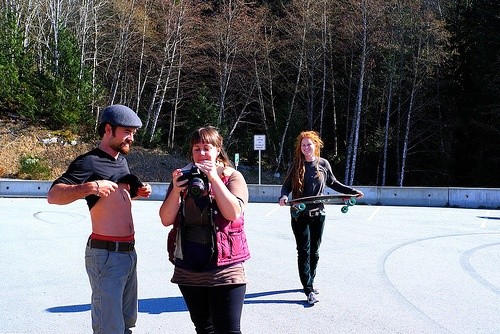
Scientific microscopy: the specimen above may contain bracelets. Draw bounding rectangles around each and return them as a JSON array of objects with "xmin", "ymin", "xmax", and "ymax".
[{"xmin": 94, "ymin": 179, "xmax": 100, "ymax": 198}]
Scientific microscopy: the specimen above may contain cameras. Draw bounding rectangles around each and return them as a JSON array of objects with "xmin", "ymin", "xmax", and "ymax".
[{"xmin": 178, "ymin": 166, "xmax": 211, "ymax": 199}]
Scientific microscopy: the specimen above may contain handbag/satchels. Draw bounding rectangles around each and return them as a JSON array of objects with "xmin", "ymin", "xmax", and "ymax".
[{"xmin": 173, "ymin": 224, "xmax": 217, "ymax": 272}]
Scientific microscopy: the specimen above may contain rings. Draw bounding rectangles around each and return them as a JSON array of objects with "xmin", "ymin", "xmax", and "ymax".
[{"xmin": 176, "ymin": 182, "xmax": 180, "ymax": 187}]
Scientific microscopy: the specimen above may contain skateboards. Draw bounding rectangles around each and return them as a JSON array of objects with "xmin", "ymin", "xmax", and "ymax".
[{"xmin": 285, "ymin": 194, "xmax": 360, "ymax": 219}]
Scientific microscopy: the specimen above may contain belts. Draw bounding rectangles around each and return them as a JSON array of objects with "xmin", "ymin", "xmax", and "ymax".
[{"xmin": 87, "ymin": 238, "xmax": 135, "ymax": 253}]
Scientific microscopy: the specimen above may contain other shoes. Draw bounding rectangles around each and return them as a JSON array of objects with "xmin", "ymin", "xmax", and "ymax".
[{"xmin": 307, "ymin": 290, "xmax": 320, "ymax": 305}]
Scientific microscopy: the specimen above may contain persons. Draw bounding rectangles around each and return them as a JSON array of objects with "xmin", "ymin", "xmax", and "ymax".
[
  {"xmin": 159, "ymin": 125, "xmax": 251, "ymax": 334},
  {"xmin": 278, "ymin": 131, "xmax": 364, "ymax": 305},
  {"xmin": 47, "ymin": 104, "xmax": 152, "ymax": 334}
]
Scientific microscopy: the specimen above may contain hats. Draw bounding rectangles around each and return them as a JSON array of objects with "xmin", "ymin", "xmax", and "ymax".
[{"xmin": 102, "ymin": 104, "xmax": 143, "ymax": 129}]
